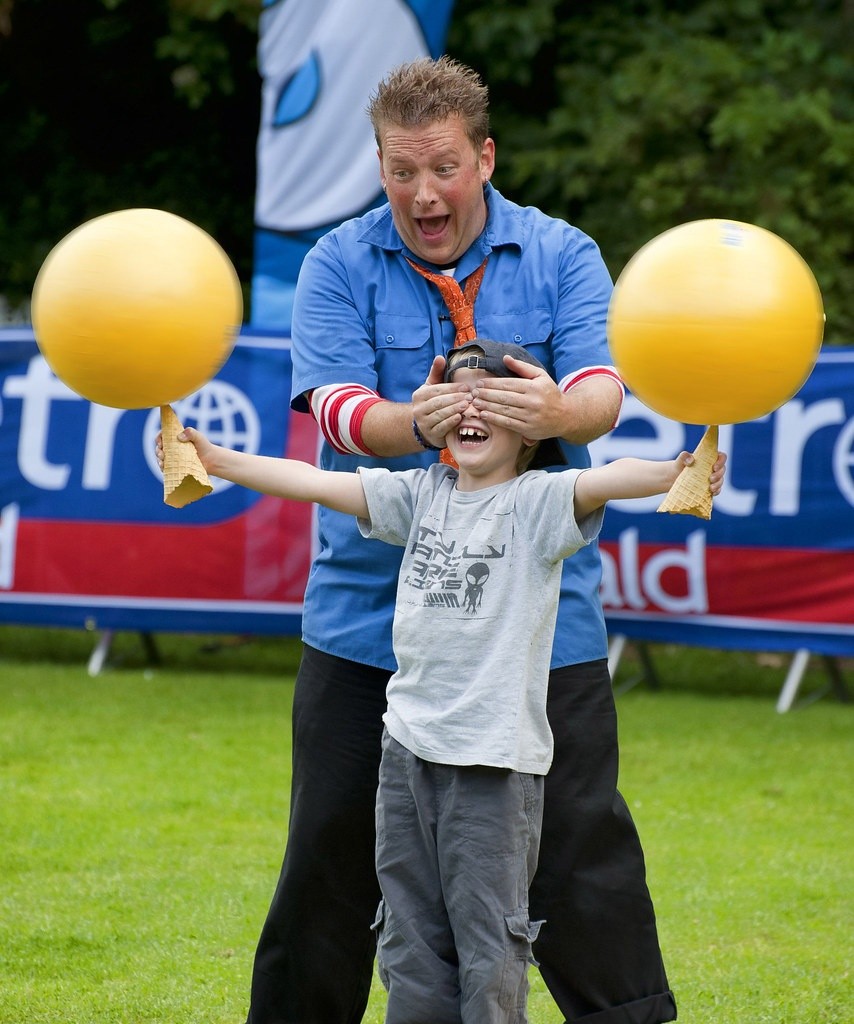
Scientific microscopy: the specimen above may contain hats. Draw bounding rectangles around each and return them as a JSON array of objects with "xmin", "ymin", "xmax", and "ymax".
[{"xmin": 443, "ymin": 339, "xmax": 569, "ymax": 465}]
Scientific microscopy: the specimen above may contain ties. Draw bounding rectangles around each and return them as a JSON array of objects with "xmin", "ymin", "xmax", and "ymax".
[{"xmin": 402, "ymin": 254, "xmax": 488, "ymax": 470}]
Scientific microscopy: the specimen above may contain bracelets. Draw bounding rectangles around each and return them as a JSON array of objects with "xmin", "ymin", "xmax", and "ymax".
[{"xmin": 412, "ymin": 419, "xmax": 447, "ymax": 453}]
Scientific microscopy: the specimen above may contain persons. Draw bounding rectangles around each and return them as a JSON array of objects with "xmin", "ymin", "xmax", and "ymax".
[
  {"xmin": 151, "ymin": 339, "xmax": 733, "ymax": 1023},
  {"xmin": 242, "ymin": 60, "xmax": 677, "ymax": 1023}
]
